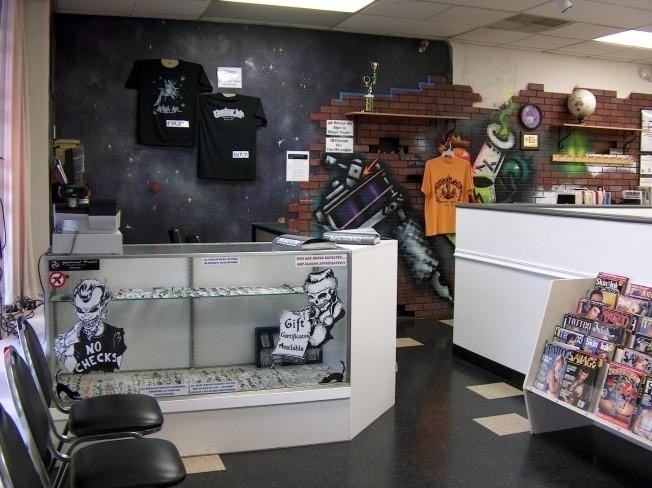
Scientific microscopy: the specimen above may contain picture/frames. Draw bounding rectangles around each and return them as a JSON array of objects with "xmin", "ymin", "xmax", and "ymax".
[{"xmin": 520, "ymin": 131, "xmax": 541, "ymax": 151}]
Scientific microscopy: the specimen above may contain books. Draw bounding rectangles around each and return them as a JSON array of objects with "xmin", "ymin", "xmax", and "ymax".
[{"xmin": 533, "ymin": 271, "xmax": 651, "ymax": 443}]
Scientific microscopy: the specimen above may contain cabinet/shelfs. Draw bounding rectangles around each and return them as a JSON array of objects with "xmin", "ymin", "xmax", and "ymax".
[
  {"xmin": 550, "ymin": 123, "xmax": 646, "ymax": 165},
  {"xmin": 47, "ymin": 222, "xmax": 400, "ymax": 453},
  {"xmin": 522, "ymin": 277, "xmax": 652, "ymax": 452}
]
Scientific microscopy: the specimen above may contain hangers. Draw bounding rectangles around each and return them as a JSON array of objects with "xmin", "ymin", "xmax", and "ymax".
[{"xmin": 439, "ymin": 141, "xmax": 458, "ymax": 159}]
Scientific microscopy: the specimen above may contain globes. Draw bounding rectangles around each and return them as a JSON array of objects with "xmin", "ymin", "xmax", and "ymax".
[{"xmin": 567, "ymin": 89, "xmax": 596, "ymax": 126}]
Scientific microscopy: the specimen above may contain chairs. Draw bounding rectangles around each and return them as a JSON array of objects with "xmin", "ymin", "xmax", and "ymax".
[
  {"xmin": 19, "ymin": 316, "xmax": 164, "ymax": 472},
  {"xmin": 168, "ymin": 225, "xmax": 187, "ymax": 243},
  {"xmin": 4, "ymin": 344, "xmax": 186, "ymax": 488},
  {"xmin": 1, "ymin": 404, "xmax": 43, "ymax": 488},
  {"xmin": 185, "ymin": 232, "xmax": 202, "ymax": 243}
]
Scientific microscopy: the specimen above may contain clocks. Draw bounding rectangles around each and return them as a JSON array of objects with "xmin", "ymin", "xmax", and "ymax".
[{"xmin": 519, "ymin": 103, "xmax": 543, "ymax": 130}]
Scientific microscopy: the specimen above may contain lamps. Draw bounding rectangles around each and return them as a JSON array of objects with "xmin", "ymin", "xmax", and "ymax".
[{"xmin": 557, "ymin": 0, "xmax": 573, "ymax": 15}]
